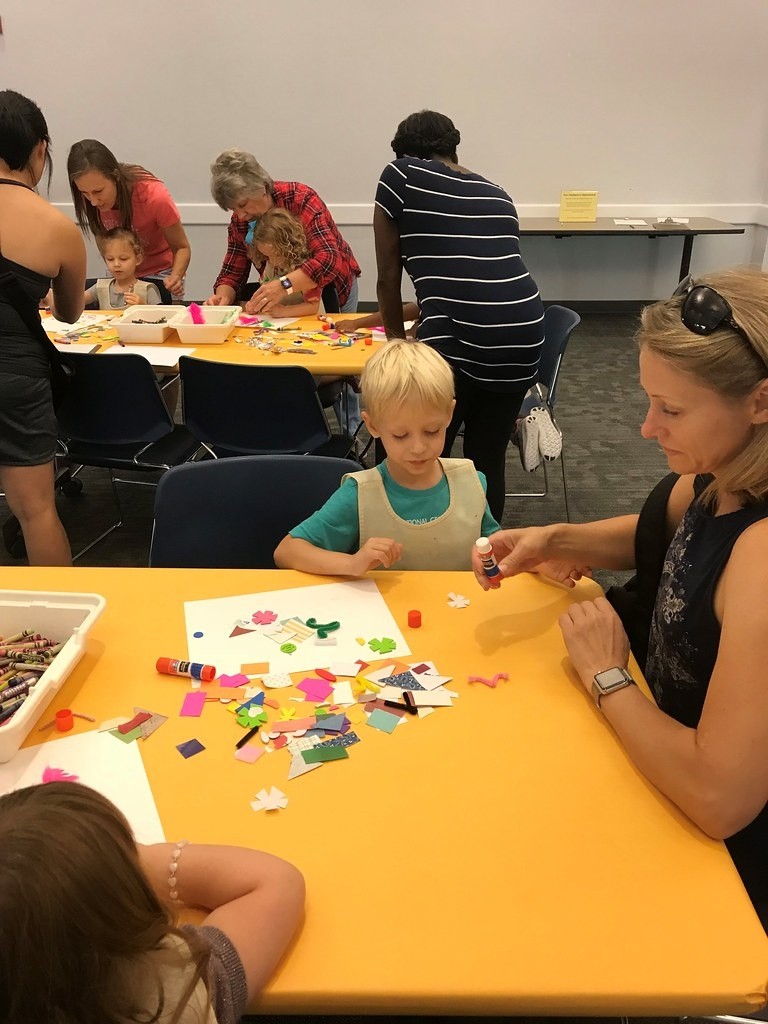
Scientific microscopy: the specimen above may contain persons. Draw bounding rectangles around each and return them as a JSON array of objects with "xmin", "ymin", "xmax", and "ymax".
[
  {"xmin": 66, "ymin": 138, "xmax": 191, "ymax": 310},
  {"xmin": 334, "ymin": 110, "xmax": 562, "ymax": 527},
  {"xmin": 273, "ymin": 338, "xmax": 594, "ymax": 589},
  {"xmin": 0, "ymin": 88, "xmax": 87, "ymax": 568},
  {"xmin": 1, "ymin": 780, "xmax": 306, "ymax": 1024},
  {"xmin": 203, "ymin": 148, "xmax": 361, "ymax": 318},
  {"xmin": 471, "ymin": 263, "xmax": 768, "ymax": 932}
]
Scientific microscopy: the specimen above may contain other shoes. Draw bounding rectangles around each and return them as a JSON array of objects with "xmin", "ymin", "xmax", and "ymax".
[
  {"xmin": 530, "ymin": 407, "xmax": 563, "ymax": 462},
  {"xmin": 518, "ymin": 415, "xmax": 540, "ymax": 472},
  {"xmin": 349, "ymin": 435, "xmax": 367, "ymax": 459}
]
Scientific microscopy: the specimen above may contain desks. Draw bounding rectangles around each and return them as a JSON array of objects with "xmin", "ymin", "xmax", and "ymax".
[
  {"xmin": 0, "ymin": 566, "xmax": 768, "ymax": 1018},
  {"xmin": 39, "ymin": 308, "xmax": 393, "ymax": 467},
  {"xmin": 518, "ymin": 216, "xmax": 745, "ymax": 296}
]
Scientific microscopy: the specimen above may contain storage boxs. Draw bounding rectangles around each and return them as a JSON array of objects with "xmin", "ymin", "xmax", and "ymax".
[
  {"xmin": 1, "ymin": 589, "xmax": 106, "ymax": 765},
  {"xmin": 109, "ymin": 303, "xmax": 242, "ymax": 344}
]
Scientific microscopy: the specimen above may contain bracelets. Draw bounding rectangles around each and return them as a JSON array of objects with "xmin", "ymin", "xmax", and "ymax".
[{"xmin": 165, "ymin": 838, "xmax": 193, "ymax": 910}]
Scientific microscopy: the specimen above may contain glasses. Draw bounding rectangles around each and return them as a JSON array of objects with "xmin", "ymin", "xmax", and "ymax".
[{"xmin": 669, "ymin": 272, "xmax": 768, "ymax": 382}]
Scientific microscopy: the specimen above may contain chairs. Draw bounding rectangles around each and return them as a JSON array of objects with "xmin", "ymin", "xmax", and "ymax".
[
  {"xmin": 47, "ymin": 277, "xmax": 365, "ymax": 569},
  {"xmin": 459, "ymin": 305, "xmax": 580, "ymax": 524}
]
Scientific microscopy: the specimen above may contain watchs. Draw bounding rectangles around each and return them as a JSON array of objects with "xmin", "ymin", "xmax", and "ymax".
[
  {"xmin": 279, "ymin": 275, "xmax": 294, "ymax": 296},
  {"xmin": 589, "ymin": 666, "xmax": 638, "ymax": 708}
]
